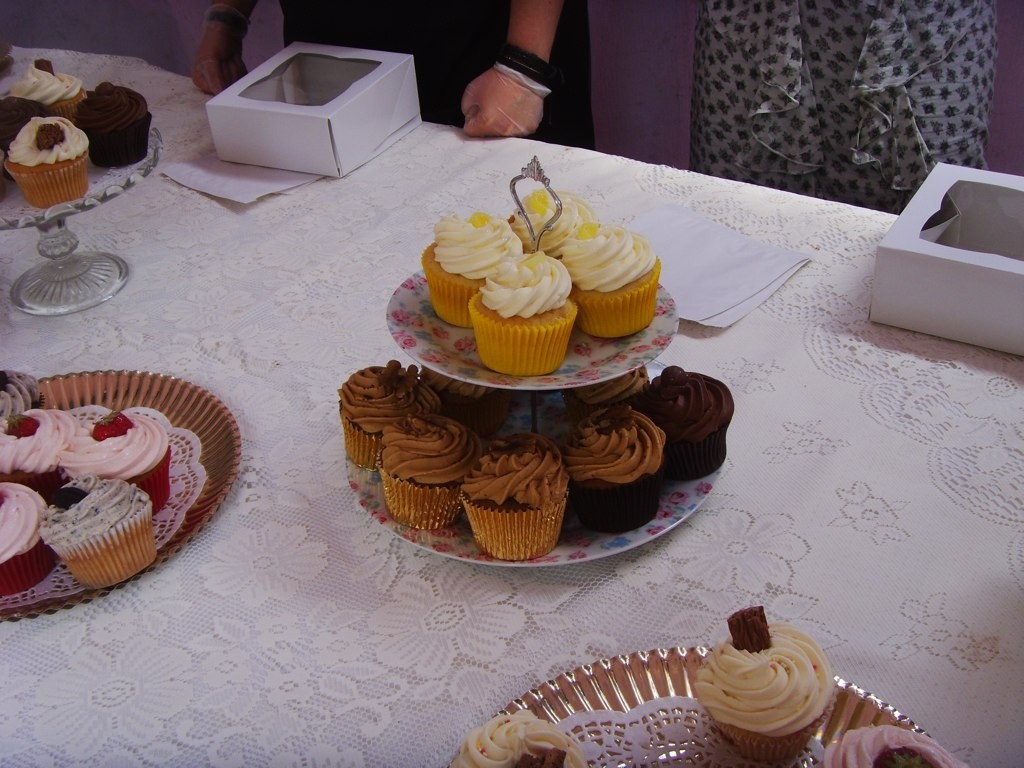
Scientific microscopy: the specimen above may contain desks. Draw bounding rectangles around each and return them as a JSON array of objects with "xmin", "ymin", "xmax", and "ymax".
[{"xmin": 0, "ymin": 44, "xmax": 1024, "ymax": 768}]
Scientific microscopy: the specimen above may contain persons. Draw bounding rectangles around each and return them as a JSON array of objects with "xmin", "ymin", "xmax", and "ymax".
[
  {"xmin": 191, "ymin": 0, "xmax": 597, "ymax": 151},
  {"xmin": 461, "ymin": 0, "xmax": 1024, "ymax": 216}
]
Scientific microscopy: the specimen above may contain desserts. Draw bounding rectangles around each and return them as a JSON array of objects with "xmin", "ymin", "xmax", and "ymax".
[
  {"xmin": 0, "ymin": 58, "xmax": 153, "ymax": 210},
  {"xmin": 809, "ymin": 724, "xmax": 971, "ymax": 768},
  {"xmin": 694, "ymin": 605, "xmax": 835, "ymax": 761},
  {"xmin": 339, "ymin": 359, "xmax": 735, "ymax": 561},
  {"xmin": 0, "ymin": 370, "xmax": 170, "ymax": 599},
  {"xmin": 421, "ymin": 188, "xmax": 661, "ymax": 376},
  {"xmin": 448, "ymin": 709, "xmax": 591, "ymax": 768}
]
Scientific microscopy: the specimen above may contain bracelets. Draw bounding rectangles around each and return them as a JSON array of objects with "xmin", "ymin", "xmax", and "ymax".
[
  {"xmin": 205, "ymin": 11, "xmax": 248, "ymax": 34},
  {"xmin": 205, "ymin": 3, "xmax": 252, "ymax": 25},
  {"xmin": 492, "ymin": 41, "xmax": 553, "ymax": 98}
]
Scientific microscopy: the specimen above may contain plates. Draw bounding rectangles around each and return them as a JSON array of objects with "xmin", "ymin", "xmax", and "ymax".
[
  {"xmin": 0, "ymin": 370, "xmax": 242, "ymax": 624},
  {"xmin": 444, "ymin": 645, "xmax": 929, "ymax": 768}
]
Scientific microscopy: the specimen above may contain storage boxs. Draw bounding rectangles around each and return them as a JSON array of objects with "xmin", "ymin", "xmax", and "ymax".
[
  {"xmin": 868, "ymin": 161, "xmax": 1024, "ymax": 357},
  {"xmin": 204, "ymin": 40, "xmax": 423, "ymax": 178}
]
